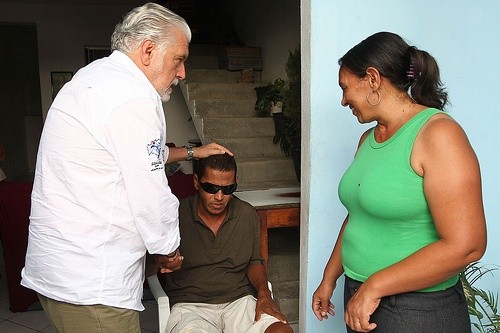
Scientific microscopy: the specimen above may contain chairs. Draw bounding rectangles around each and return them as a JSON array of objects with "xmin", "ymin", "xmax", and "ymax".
[
  {"xmin": 147, "ymin": 274, "xmax": 273, "ymax": 333},
  {"xmin": 0, "ymin": 183, "xmax": 44, "ymax": 313}
]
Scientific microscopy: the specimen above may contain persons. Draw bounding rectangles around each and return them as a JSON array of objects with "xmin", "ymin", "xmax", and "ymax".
[
  {"xmin": 18, "ymin": 3, "xmax": 233, "ymax": 333},
  {"xmin": 312, "ymin": 31, "xmax": 487, "ymax": 333},
  {"xmin": 144, "ymin": 155, "xmax": 296, "ymax": 333}
]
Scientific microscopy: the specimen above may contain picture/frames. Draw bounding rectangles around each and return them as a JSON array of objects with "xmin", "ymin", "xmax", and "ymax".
[{"xmin": 51, "ymin": 71, "xmax": 73, "ymax": 102}]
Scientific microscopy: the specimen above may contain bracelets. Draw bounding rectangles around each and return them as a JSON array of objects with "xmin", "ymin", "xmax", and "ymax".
[{"xmin": 166, "ymin": 250, "xmax": 177, "ymax": 258}]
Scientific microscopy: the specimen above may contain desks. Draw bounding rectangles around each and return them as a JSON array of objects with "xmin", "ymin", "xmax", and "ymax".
[{"xmin": 233, "ymin": 188, "xmax": 301, "ymax": 274}]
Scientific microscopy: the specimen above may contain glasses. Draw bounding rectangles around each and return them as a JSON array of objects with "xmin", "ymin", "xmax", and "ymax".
[{"xmin": 198, "ymin": 180, "xmax": 237, "ymax": 195}]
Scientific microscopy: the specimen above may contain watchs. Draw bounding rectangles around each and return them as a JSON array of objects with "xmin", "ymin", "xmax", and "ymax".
[{"xmin": 185, "ymin": 145, "xmax": 194, "ymax": 161}]
